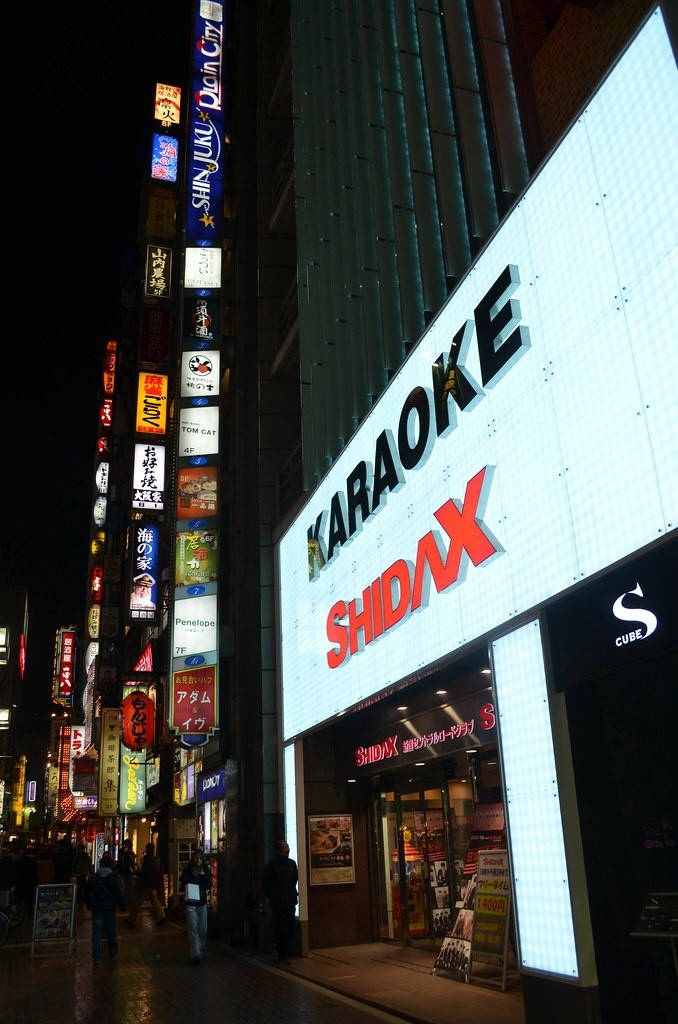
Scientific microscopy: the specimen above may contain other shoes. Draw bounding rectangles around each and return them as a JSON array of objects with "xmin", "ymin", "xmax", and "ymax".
[
  {"xmin": 109, "ymin": 944, "xmax": 120, "ymax": 960},
  {"xmin": 153, "ymin": 917, "xmax": 168, "ymax": 927},
  {"xmin": 92, "ymin": 958, "xmax": 101, "ymax": 964},
  {"xmin": 122, "ymin": 919, "xmax": 134, "ymax": 928},
  {"xmin": 190, "ymin": 947, "xmax": 204, "ymax": 963},
  {"xmin": 280, "ymin": 959, "xmax": 292, "ymax": 966},
  {"xmin": 271, "ymin": 943, "xmax": 279, "ymax": 957}
]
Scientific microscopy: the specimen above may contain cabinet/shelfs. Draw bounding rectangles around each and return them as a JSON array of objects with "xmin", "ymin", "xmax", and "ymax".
[{"xmin": 175, "ymin": 836, "xmax": 197, "ymax": 895}]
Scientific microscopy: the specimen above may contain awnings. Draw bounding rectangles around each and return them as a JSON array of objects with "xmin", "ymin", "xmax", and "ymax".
[{"xmin": 127, "ymin": 800, "xmax": 168, "ymax": 819}]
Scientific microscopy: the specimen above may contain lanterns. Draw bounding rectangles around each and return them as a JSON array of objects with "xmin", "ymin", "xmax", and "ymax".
[{"xmin": 118, "ymin": 685, "xmax": 155, "ymax": 753}]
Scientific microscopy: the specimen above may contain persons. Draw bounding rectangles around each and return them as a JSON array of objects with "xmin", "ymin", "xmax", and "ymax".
[
  {"xmin": 437, "ymin": 890, "xmax": 449, "ymax": 908},
  {"xmin": 435, "ymin": 940, "xmax": 469, "ymax": 971},
  {"xmin": 0, "ymin": 833, "xmax": 135, "ymax": 920},
  {"xmin": 438, "ymin": 862, "xmax": 448, "ymax": 886},
  {"xmin": 179, "ymin": 849, "xmax": 212, "ymax": 963},
  {"xmin": 435, "ymin": 911, "xmax": 451, "ymax": 938},
  {"xmin": 124, "ymin": 843, "xmax": 168, "ymax": 927},
  {"xmin": 83, "ymin": 851, "xmax": 127, "ymax": 964},
  {"xmin": 262, "ymin": 840, "xmax": 298, "ymax": 966},
  {"xmin": 455, "ymin": 862, "xmax": 464, "ymax": 883}
]
